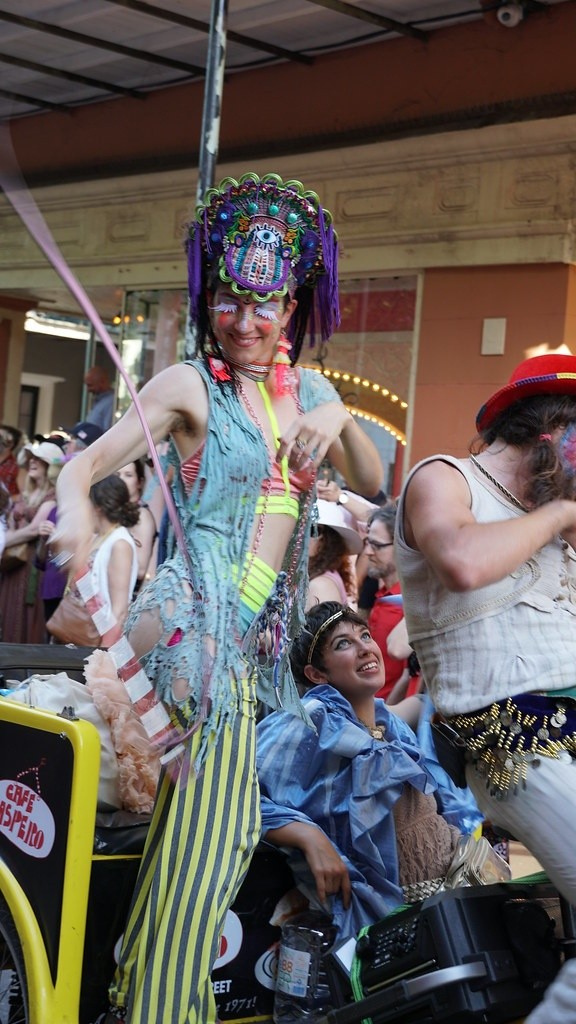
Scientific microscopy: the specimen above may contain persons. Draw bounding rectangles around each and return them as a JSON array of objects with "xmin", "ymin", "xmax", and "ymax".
[
  {"xmin": 394, "ymin": 355, "xmax": 576, "ymax": 1024},
  {"xmin": 300, "ymin": 479, "xmax": 424, "ymax": 704},
  {"xmin": 52, "ymin": 173, "xmax": 381, "ymax": 1024},
  {"xmin": 254, "ymin": 601, "xmax": 481, "ymax": 950},
  {"xmin": 0, "ymin": 420, "xmax": 181, "ymax": 648}
]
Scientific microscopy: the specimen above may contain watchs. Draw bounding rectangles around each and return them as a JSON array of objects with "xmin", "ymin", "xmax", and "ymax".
[{"xmin": 337, "ymin": 492, "xmax": 348, "ymax": 506}]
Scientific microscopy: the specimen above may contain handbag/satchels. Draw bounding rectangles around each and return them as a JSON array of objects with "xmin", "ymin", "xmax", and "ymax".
[
  {"xmin": 45, "ymin": 590, "xmax": 103, "ymax": 648},
  {"xmin": 32, "ymin": 535, "xmax": 49, "ymax": 571},
  {"xmin": 6, "ymin": 672, "xmax": 124, "ymax": 811}
]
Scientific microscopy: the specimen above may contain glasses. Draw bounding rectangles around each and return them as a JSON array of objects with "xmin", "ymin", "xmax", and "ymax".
[
  {"xmin": 311, "ymin": 526, "xmax": 322, "ymax": 537},
  {"xmin": 363, "ymin": 537, "xmax": 393, "ymax": 550}
]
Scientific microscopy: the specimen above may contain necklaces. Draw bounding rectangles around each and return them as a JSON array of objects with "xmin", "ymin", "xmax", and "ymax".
[
  {"xmin": 262, "ymin": 435, "xmax": 315, "ymax": 659},
  {"xmin": 469, "ymin": 454, "xmax": 530, "ymax": 513},
  {"xmin": 228, "ymin": 353, "xmax": 307, "ymax": 595}
]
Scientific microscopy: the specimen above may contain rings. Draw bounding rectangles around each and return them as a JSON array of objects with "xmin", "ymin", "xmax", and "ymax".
[{"xmin": 297, "ymin": 438, "xmax": 307, "ymax": 449}]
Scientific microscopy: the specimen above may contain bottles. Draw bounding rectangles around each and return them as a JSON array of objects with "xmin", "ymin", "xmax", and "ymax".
[{"xmin": 273, "ymin": 902, "xmax": 339, "ymax": 1024}]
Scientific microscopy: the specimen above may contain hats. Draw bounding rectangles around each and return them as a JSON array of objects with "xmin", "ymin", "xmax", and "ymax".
[
  {"xmin": 42, "ymin": 430, "xmax": 71, "ymax": 442},
  {"xmin": 183, "ymin": 172, "xmax": 341, "ymax": 368},
  {"xmin": 34, "ymin": 434, "xmax": 65, "ymax": 446},
  {"xmin": 24, "ymin": 442, "xmax": 64, "ymax": 464},
  {"xmin": 63, "ymin": 422, "xmax": 104, "ymax": 446},
  {"xmin": 316, "ymin": 498, "xmax": 364, "ymax": 555},
  {"xmin": 475, "ymin": 354, "xmax": 576, "ymax": 435}
]
